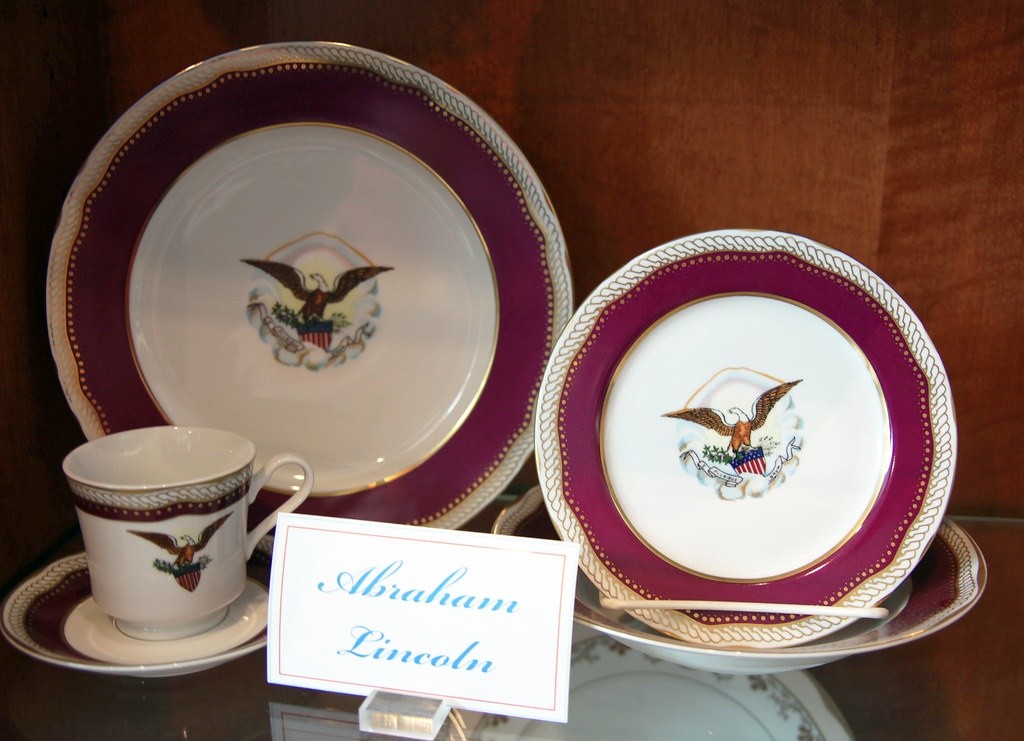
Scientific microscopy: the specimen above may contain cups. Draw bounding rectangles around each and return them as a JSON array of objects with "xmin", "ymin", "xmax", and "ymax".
[{"xmin": 64, "ymin": 428, "xmax": 313, "ymax": 641}]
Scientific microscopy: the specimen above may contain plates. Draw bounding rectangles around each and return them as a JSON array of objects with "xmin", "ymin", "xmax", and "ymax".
[
  {"xmin": 534, "ymin": 229, "xmax": 958, "ymax": 649},
  {"xmin": 491, "ymin": 484, "xmax": 990, "ymax": 675},
  {"xmin": 465, "ymin": 635, "xmax": 857, "ymax": 741},
  {"xmin": 0, "ymin": 553, "xmax": 269, "ymax": 679},
  {"xmin": 47, "ymin": 42, "xmax": 569, "ymax": 553}
]
